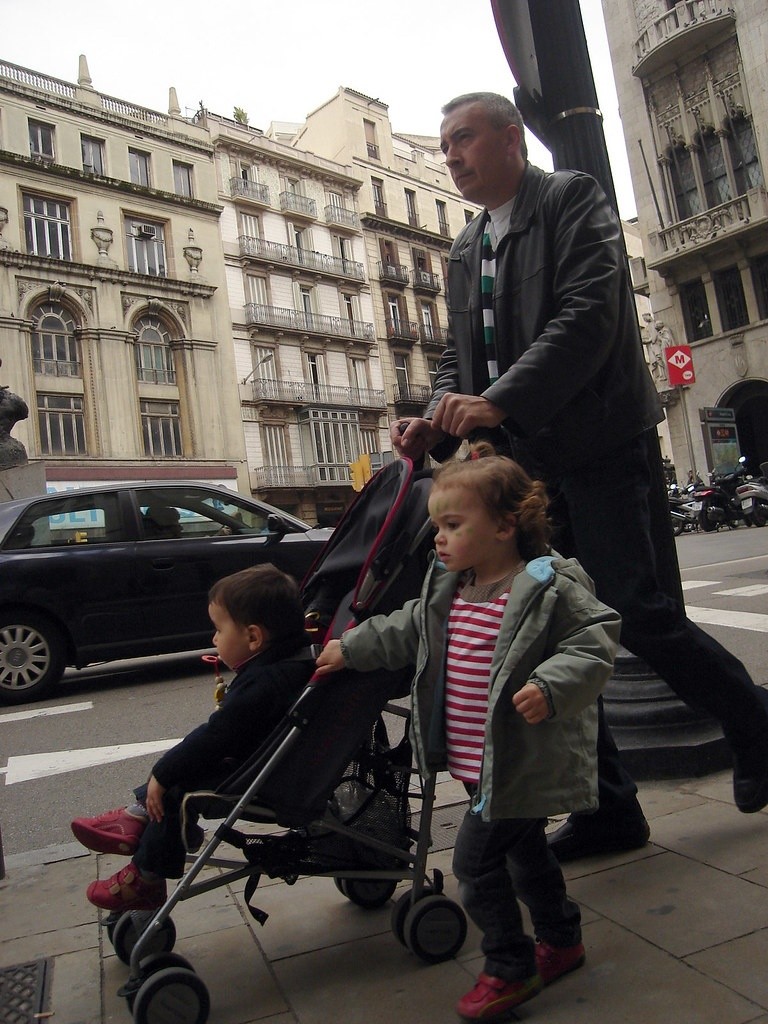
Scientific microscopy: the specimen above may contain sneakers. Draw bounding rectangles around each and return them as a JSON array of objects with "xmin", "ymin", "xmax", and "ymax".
[
  {"xmin": 454, "ymin": 970, "xmax": 545, "ymax": 1021},
  {"xmin": 535, "ymin": 936, "xmax": 587, "ymax": 980},
  {"xmin": 70, "ymin": 806, "xmax": 149, "ymax": 856},
  {"xmin": 86, "ymin": 859, "xmax": 168, "ymax": 910}
]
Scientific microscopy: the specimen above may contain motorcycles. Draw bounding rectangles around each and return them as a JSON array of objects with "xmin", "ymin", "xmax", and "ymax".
[{"xmin": 667, "ymin": 455, "xmax": 768, "ymax": 535}]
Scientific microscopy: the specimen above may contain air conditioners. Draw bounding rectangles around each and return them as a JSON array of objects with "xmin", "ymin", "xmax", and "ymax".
[
  {"xmin": 387, "ymin": 266, "xmax": 396, "ymax": 277},
  {"xmin": 419, "ymin": 272, "xmax": 430, "ymax": 284},
  {"xmin": 138, "ymin": 225, "xmax": 155, "ymax": 238}
]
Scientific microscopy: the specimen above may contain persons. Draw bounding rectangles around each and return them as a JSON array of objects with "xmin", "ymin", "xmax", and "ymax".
[
  {"xmin": 315, "ymin": 442, "xmax": 622, "ymax": 1021},
  {"xmin": 685, "ymin": 469, "xmax": 704, "ymax": 487},
  {"xmin": 70, "ymin": 561, "xmax": 323, "ymax": 912},
  {"xmin": 390, "ymin": 94, "xmax": 768, "ymax": 861}
]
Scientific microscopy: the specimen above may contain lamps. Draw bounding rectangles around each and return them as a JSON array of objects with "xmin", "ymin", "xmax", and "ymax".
[{"xmin": 242, "ymin": 353, "xmax": 273, "ymax": 385}]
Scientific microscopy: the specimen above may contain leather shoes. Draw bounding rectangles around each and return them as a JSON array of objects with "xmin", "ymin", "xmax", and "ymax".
[
  {"xmin": 722, "ymin": 686, "xmax": 768, "ymax": 814},
  {"xmin": 546, "ymin": 796, "xmax": 651, "ymax": 860}
]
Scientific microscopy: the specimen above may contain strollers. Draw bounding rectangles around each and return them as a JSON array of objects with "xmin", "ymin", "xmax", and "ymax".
[{"xmin": 101, "ymin": 408, "xmax": 467, "ymax": 1024}]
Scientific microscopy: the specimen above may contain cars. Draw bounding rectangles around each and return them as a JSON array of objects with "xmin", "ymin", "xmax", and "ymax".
[{"xmin": 0, "ymin": 480, "xmax": 340, "ymax": 704}]
